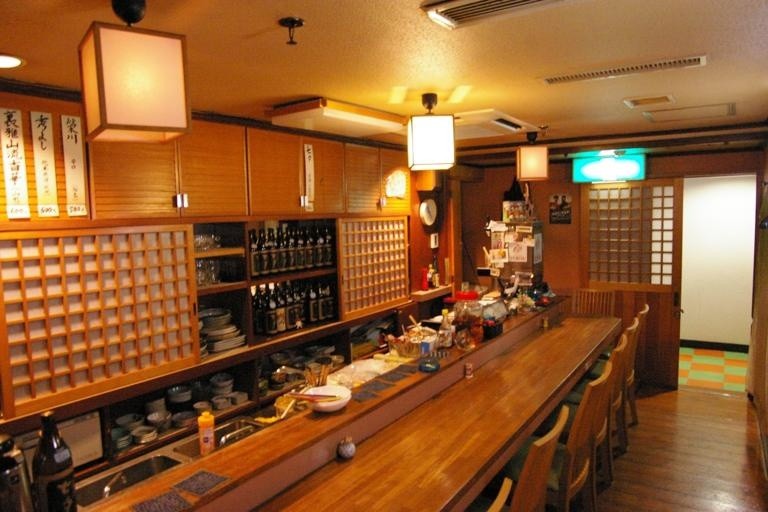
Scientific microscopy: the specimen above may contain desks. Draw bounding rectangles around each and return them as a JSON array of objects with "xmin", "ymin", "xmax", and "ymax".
[{"xmin": 253, "ymin": 312, "xmax": 622, "ymax": 512}]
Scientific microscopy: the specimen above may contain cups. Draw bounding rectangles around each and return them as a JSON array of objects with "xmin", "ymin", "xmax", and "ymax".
[{"xmin": 195, "ymin": 233, "xmax": 220, "ymax": 286}]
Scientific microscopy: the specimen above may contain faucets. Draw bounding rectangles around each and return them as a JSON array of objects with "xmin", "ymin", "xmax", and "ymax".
[
  {"xmin": 102, "ymin": 470, "xmax": 127, "ymax": 499},
  {"xmin": 219, "ymin": 427, "xmax": 255, "ymax": 446}
]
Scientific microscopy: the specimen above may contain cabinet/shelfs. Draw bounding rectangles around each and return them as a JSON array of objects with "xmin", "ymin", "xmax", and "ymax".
[
  {"xmin": 489, "ymin": 220, "xmax": 543, "ymax": 286},
  {"xmin": 12, "ymin": 105, "xmax": 410, "ymax": 493}
]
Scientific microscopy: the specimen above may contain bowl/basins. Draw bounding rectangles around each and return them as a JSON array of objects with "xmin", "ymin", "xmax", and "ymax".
[
  {"xmin": 111, "ymin": 373, "xmax": 249, "ymax": 452},
  {"xmin": 270, "ymin": 353, "xmax": 290, "ymax": 366},
  {"xmin": 304, "ymin": 384, "xmax": 352, "ymax": 413}
]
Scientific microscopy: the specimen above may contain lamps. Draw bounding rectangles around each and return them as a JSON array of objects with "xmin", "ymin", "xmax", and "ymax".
[
  {"xmin": 517, "ymin": 130, "xmax": 550, "ymax": 182},
  {"xmin": 406, "ymin": 92, "xmax": 456, "ymax": 172},
  {"xmin": 268, "ymin": 96, "xmax": 406, "ymax": 139},
  {"xmin": 77, "ymin": 0, "xmax": 192, "ymax": 144}
]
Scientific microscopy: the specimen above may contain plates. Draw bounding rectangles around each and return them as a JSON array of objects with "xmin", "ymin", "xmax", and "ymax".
[{"xmin": 198, "ymin": 308, "xmax": 246, "ymax": 360}]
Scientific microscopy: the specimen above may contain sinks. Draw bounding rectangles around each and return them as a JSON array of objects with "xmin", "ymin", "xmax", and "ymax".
[
  {"xmin": 75, "ymin": 450, "xmax": 189, "ymax": 506},
  {"xmin": 172, "ymin": 418, "xmax": 271, "ymax": 456}
]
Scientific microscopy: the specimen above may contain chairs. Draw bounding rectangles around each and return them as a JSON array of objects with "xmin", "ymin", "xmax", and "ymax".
[{"xmin": 486, "ymin": 286, "xmax": 651, "ymax": 512}]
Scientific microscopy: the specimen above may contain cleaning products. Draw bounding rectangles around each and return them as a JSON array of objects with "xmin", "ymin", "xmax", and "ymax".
[{"xmin": 197, "ymin": 410, "xmax": 216, "ymax": 454}]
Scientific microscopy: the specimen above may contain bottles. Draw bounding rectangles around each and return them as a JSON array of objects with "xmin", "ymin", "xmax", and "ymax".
[
  {"xmin": 421, "ymin": 255, "xmax": 440, "ymax": 291},
  {"xmin": 250, "ymin": 224, "xmax": 334, "ymax": 337},
  {"xmin": 438, "ymin": 309, "xmax": 453, "ymax": 349},
  {"xmin": 0, "ymin": 411, "xmax": 77, "ymax": 512}
]
